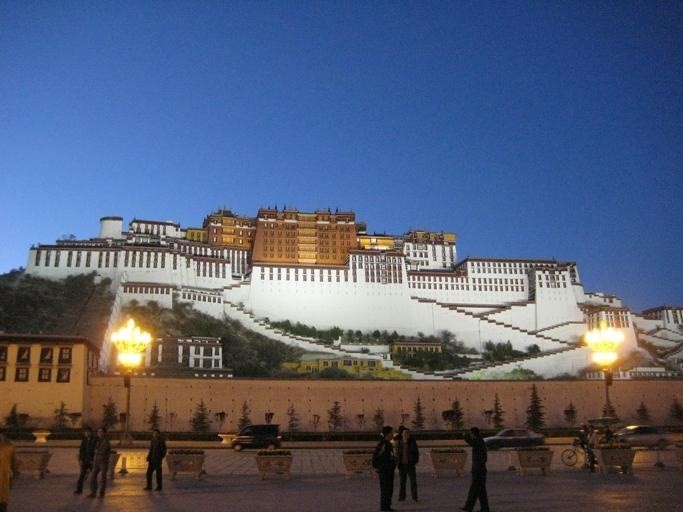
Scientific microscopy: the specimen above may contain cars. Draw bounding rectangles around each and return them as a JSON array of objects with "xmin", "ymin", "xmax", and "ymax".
[
  {"xmin": 599, "ymin": 425, "xmax": 673, "ymax": 449},
  {"xmin": 483, "ymin": 428, "xmax": 545, "ymax": 451}
]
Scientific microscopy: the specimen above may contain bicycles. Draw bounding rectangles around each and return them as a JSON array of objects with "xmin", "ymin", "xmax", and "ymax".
[{"xmin": 561, "ymin": 437, "xmax": 599, "ymax": 466}]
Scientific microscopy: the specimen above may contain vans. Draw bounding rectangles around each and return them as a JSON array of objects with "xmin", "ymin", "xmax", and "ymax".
[{"xmin": 231, "ymin": 423, "xmax": 282, "ymax": 452}]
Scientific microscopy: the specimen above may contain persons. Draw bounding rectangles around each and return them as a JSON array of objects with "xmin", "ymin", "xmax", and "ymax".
[
  {"xmin": 396, "ymin": 427, "xmax": 422, "ymax": 503},
  {"xmin": 142, "ymin": 429, "xmax": 167, "ymax": 491},
  {"xmin": 459, "ymin": 426, "xmax": 490, "ymax": 512},
  {"xmin": 398, "ymin": 425, "xmax": 416, "ymax": 444},
  {"xmin": 0, "ymin": 428, "xmax": 19, "ymax": 512},
  {"xmin": 72, "ymin": 427, "xmax": 98, "ymax": 495},
  {"xmin": 87, "ymin": 427, "xmax": 111, "ymax": 499},
  {"xmin": 572, "ymin": 422, "xmax": 614, "ymax": 472},
  {"xmin": 372, "ymin": 425, "xmax": 397, "ymax": 512}
]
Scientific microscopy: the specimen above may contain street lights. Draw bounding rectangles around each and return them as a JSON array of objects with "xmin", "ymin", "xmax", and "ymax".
[
  {"xmin": 111, "ymin": 319, "xmax": 153, "ymax": 446},
  {"xmin": 583, "ymin": 320, "xmax": 625, "ymax": 415}
]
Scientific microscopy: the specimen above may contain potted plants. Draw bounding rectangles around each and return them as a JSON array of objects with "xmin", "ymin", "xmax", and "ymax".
[
  {"xmin": 342, "ymin": 449, "xmax": 380, "ymax": 479},
  {"xmin": 596, "ymin": 439, "xmax": 636, "ymax": 474},
  {"xmin": 165, "ymin": 448, "xmax": 206, "ymax": 480},
  {"xmin": 509, "ymin": 448, "xmax": 553, "ymax": 478},
  {"xmin": 428, "ymin": 449, "xmax": 469, "ymax": 479},
  {"xmin": 254, "ymin": 450, "xmax": 293, "ymax": 479}
]
